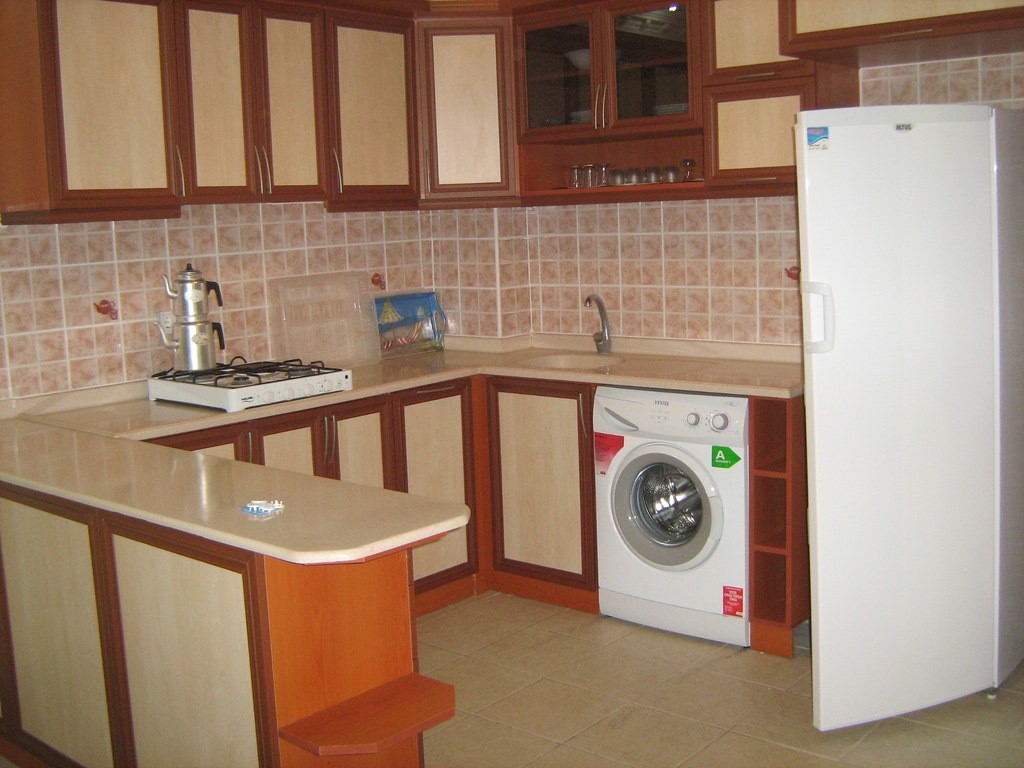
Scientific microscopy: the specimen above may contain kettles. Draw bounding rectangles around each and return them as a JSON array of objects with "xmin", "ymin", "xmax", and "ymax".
[
  {"xmin": 152, "ymin": 316, "xmax": 225, "ymax": 372},
  {"xmin": 162, "ymin": 263, "xmax": 223, "ymax": 315}
]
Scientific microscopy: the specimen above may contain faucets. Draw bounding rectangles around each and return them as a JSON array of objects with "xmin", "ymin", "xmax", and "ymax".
[{"xmin": 583, "ymin": 294, "xmax": 612, "ymax": 353}]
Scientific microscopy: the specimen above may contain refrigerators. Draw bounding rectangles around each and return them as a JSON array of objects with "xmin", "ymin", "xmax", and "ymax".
[{"xmin": 791, "ymin": 102, "xmax": 1024, "ymax": 732}]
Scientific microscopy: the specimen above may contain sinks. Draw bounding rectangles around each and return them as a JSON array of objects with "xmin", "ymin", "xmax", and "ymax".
[{"xmin": 520, "ymin": 354, "xmax": 622, "ymax": 370}]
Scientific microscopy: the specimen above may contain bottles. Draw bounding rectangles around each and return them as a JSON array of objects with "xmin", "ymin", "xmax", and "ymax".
[
  {"xmin": 570, "ymin": 163, "xmax": 608, "ymax": 187},
  {"xmin": 681, "ymin": 159, "xmax": 696, "ymax": 181},
  {"xmin": 649, "ymin": 103, "xmax": 688, "ymax": 116}
]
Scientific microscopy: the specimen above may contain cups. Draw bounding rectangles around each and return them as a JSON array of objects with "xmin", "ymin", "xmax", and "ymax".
[{"xmin": 612, "ymin": 167, "xmax": 678, "ymax": 186}]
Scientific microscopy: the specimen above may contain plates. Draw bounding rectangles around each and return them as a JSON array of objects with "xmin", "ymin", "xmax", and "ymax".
[{"xmin": 567, "ymin": 109, "xmax": 592, "ymax": 124}]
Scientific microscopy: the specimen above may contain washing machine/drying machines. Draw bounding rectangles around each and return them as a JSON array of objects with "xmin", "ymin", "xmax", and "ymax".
[{"xmin": 592, "ymin": 384, "xmax": 750, "ymax": 647}]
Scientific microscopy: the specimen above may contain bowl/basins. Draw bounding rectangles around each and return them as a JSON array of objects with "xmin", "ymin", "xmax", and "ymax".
[{"xmin": 564, "ymin": 48, "xmax": 620, "ymax": 70}]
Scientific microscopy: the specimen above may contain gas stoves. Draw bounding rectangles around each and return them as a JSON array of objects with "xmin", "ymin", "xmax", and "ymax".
[{"xmin": 148, "ymin": 356, "xmax": 353, "ymax": 413}]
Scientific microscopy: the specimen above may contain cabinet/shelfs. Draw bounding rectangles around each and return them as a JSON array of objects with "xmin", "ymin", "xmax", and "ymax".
[
  {"xmin": 1, "ymin": 0, "xmax": 1024, "ymax": 227},
  {"xmin": 0, "ymin": 377, "xmax": 809, "ymax": 768}
]
